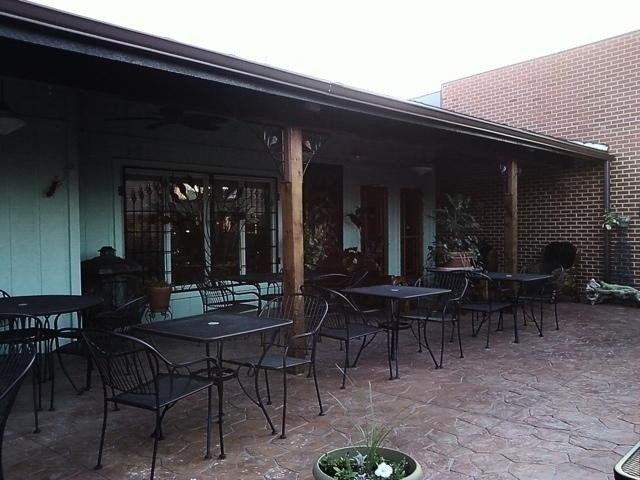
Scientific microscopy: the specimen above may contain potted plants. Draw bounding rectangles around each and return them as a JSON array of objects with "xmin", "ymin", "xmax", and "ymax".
[
  {"xmin": 418, "ymin": 190, "xmax": 482, "ymax": 269},
  {"xmin": 313, "ymin": 382, "xmax": 422, "ymax": 480}
]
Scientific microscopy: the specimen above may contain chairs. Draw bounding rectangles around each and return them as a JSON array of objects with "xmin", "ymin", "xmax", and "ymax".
[
  {"xmin": 520, "ymin": 240, "xmax": 579, "ymax": 306},
  {"xmin": 1, "ymin": 254, "xmax": 151, "ymax": 436},
  {"xmin": 81, "ymin": 327, "xmax": 223, "ymax": 480},
  {"xmin": 190, "ymin": 253, "xmax": 563, "ymax": 390},
  {"xmin": 219, "ymin": 294, "xmax": 327, "ymax": 438}
]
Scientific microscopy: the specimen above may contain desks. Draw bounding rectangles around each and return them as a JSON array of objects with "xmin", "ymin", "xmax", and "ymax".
[{"xmin": 131, "ymin": 311, "xmax": 294, "ymax": 458}]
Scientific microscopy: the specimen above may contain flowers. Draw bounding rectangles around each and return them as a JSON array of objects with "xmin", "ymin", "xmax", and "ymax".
[{"xmin": 601, "ymin": 208, "xmax": 631, "ymax": 233}]
